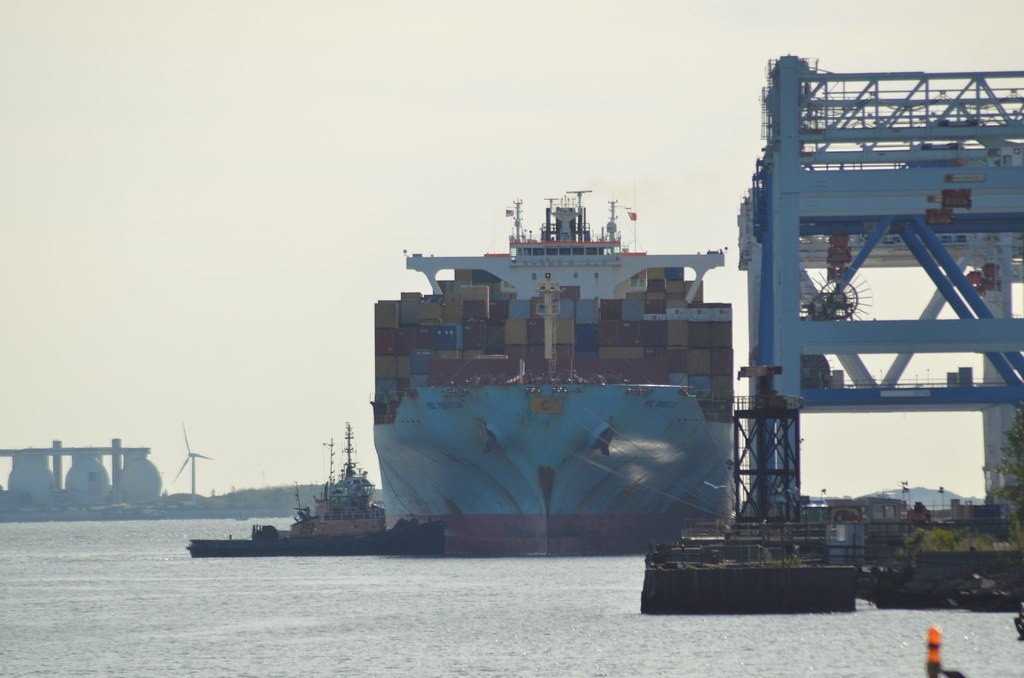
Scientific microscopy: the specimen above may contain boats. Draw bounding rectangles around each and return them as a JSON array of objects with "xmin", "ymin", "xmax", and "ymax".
[
  {"xmin": 186, "ymin": 516, "xmax": 446, "ymax": 559},
  {"xmin": 286, "ymin": 420, "xmax": 386, "ymax": 535}
]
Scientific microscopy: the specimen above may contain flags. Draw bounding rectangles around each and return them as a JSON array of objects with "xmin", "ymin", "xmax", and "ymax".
[
  {"xmin": 505, "ymin": 210, "xmax": 515, "ymax": 217},
  {"xmin": 628, "ymin": 212, "xmax": 637, "ymax": 221}
]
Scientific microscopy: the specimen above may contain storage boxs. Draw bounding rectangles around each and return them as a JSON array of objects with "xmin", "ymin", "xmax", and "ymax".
[{"xmin": 374, "ymin": 266, "xmax": 734, "ymax": 425}]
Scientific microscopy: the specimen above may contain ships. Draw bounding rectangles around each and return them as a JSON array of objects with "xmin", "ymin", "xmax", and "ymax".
[{"xmin": 370, "ymin": 190, "xmax": 736, "ymax": 559}]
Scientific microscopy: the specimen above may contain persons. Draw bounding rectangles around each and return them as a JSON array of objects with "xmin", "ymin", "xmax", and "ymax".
[{"xmin": 447, "ymin": 362, "xmax": 666, "ymax": 388}]
